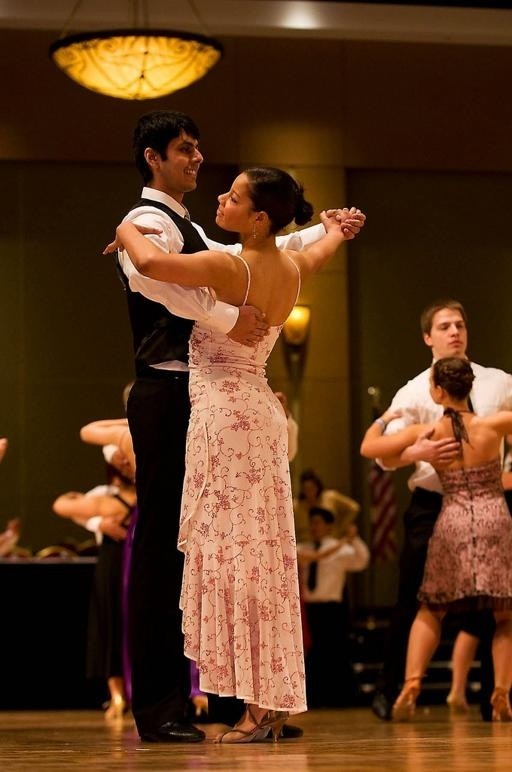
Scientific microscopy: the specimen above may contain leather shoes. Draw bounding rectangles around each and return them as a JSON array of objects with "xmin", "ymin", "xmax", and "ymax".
[
  {"xmin": 364, "ymin": 686, "xmax": 389, "ymax": 718},
  {"xmin": 142, "ymin": 723, "xmax": 205, "ymax": 742},
  {"xmin": 206, "ymin": 710, "xmax": 304, "ymax": 739}
]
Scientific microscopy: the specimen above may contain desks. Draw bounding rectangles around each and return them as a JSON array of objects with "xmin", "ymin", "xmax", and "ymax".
[{"xmin": 1, "ymin": 554, "xmax": 98, "ymax": 711}]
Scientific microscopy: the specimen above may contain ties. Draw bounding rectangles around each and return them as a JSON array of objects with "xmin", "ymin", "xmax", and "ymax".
[{"xmin": 308, "ymin": 542, "xmax": 320, "ymax": 590}]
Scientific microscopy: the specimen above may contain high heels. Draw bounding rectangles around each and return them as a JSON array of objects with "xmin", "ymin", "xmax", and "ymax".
[
  {"xmin": 213, "ymin": 710, "xmax": 289, "ymax": 743},
  {"xmin": 392, "ymin": 675, "xmax": 422, "ymax": 719},
  {"xmin": 491, "ymin": 688, "xmax": 512, "ymax": 722},
  {"xmin": 104, "ymin": 694, "xmax": 126, "ymax": 718}
]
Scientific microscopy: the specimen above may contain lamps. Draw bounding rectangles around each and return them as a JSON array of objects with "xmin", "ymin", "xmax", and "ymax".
[
  {"xmin": 281, "ymin": 303, "xmax": 311, "ymax": 381},
  {"xmin": 50, "ymin": 1, "xmax": 228, "ymax": 101}
]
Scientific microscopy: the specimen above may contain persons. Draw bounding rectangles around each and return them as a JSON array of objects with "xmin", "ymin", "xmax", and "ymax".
[
  {"xmin": 115, "ymin": 110, "xmax": 368, "ymax": 738},
  {"xmin": 100, "ymin": 165, "xmax": 364, "ymax": 741},
  {"xmin": 296, "ymin": 505, "xmax": 370, "ymax": 710},
  {"xmin": 274, "ymin": 391, "xmax": 299, "ymax": 462},
  {"xmin": 360, "ymin": 300, "xmax": 511, "ymax": 721},
  {"xmin": 53, "ymin": 382, "xmax": 208, "ymax": 720},
  {"xmin": 292, "ymin": 466, "xmax": 360, "ymax": 542}
]
